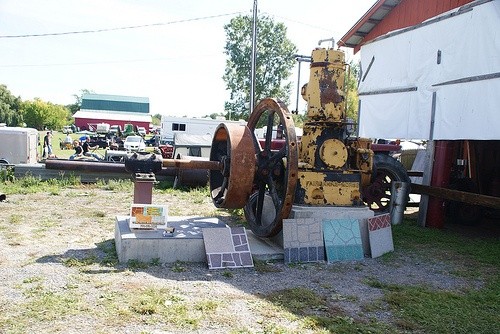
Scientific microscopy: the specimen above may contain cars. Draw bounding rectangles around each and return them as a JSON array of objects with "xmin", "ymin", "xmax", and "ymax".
[
  {"xmin": 88, "ymin": 125, "xmax": 127, "ymax": 151},
  {"xmin": 143, "ymin": 125, "xmax": 174, "ymax": 159},
  {"xmin": 62, "ymin": 126, "xmax": 74, "ymax": 134},
  {"xmin": 122, "ymin": 136, "xmax": 147, "ymax": 154},
  {"xmin": 137, "ymin": 127, "xmax": 147, "ymax": 137}
]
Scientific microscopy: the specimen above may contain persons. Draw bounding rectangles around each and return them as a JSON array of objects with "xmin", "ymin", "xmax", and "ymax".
[
  {"xmin": 151, "ymin": 129, "xmax": 158, "ymax": 146},
  {"xmin": 112, "ymin": 138, "xmax": 124, "ymax": 145},
  {"xmin": 43, "ymin": 132, "xmax": 52, "ymax": 154},
  {"xmin": 70, "ymin": 141, "xmax": 83, "ymax": 159},
  {"xmin": 82, "ymin": 137, "xmax": 102, "ymax": 161},
  {"xmin": 62, "ymin": 133, "xmax": 71, "ymax": 150}
]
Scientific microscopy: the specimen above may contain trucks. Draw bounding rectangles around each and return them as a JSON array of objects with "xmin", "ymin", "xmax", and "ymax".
[{"xmin": 159, "ymin": 115, "xmax": 248, "ymax": 146}]
